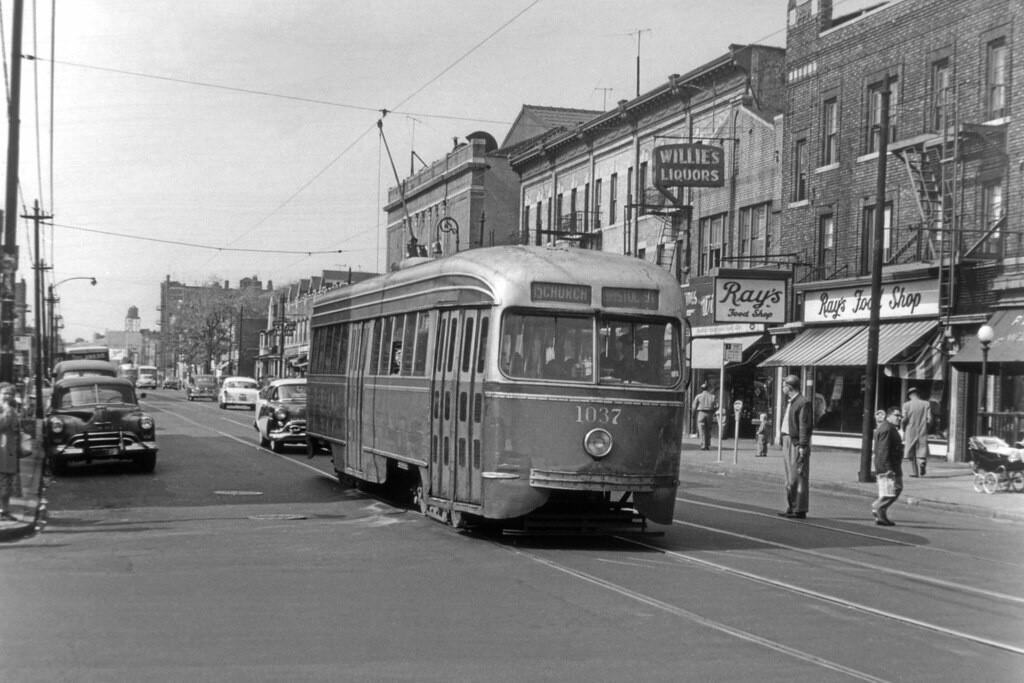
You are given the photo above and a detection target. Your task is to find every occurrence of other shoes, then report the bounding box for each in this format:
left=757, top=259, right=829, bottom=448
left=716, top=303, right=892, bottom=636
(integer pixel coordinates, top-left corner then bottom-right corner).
left=779, top=511, right=792, bottom=517
left=786, top=512, right=805, bottom=519
left=876, top=517, right=895, bottom=527
left=872, top=509, right=888, bottom=525
left=910, top=474, right=917, bottom=477
left=921, top=466, right=925, bottom=475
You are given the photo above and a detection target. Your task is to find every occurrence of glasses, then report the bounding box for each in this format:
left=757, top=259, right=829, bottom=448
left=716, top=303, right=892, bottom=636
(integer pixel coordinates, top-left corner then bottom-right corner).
left=888, top=413, right=902, bottom=418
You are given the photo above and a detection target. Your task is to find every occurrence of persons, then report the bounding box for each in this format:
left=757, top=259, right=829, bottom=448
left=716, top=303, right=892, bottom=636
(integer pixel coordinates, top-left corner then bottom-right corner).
left=871, top=406, right=905, bottom=525
left=756, top=412, right=769, bottom=456
left=777, top=374, right=813, bottom=519
left=692, top=383, right=715, bottom=449
left=902, top=387, right=932, bottom=476
left=392, top=343, right=402, bottom=376
left=615, top=333, right=653, bottom=382
left=0, top=377, right=36, bottom=514
left=714, top=385, right=730, bottom=437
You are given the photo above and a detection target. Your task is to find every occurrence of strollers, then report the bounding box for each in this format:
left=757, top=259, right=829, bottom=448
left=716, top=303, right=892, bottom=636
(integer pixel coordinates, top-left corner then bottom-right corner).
left=968, top=435, right=1024, bottom=495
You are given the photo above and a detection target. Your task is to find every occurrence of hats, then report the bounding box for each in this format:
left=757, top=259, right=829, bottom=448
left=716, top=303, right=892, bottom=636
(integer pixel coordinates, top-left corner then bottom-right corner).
left=619, top=333, right=643, bottom=350
left=906, top=387, right=921, bottom=400
left=783, top=375, right=802, bottom=389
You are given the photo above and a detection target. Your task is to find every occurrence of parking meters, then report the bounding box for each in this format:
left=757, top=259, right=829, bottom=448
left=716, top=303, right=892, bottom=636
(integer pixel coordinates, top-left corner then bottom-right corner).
left=733, top=400, right=743, bottom=464
left=876, top=409, right=886, bottom=433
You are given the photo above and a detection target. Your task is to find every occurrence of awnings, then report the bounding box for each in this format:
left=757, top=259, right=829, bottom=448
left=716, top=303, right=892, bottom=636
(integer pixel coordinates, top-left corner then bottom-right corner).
left=664, top=335, right=763, bottom=369
left=948, top=308, right=1023, bottom=375
left=757, top=320, right=938, bottom=367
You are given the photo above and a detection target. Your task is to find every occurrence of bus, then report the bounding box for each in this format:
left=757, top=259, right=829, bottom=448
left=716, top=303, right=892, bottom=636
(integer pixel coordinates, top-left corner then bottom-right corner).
left=305, top=242, right=693, bottom=539
left=139, top=366, right=157, bottom=375
left=60, top=346, right=111, bottom=361
left=119, top=365, right=138, bottom=381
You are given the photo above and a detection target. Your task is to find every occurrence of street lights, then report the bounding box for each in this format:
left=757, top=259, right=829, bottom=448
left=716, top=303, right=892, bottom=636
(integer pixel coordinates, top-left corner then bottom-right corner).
left=978, top=326, right=993, bottom=431
left=433, top=218, right=460, bottom=255
left=48, top=277, right=98, bottom=373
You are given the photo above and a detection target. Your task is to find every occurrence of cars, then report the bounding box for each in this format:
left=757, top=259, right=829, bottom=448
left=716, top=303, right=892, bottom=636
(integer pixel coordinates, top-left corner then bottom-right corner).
left=162, top=376, right=180, bottom=390
left=137, top=374, right=157, bottom=389
left=44, top=361, right=117, bottom=401
left=185, top=374, right=219, bottom=402
left=42, top=378, right=156, bottom=475
left=218, top=376, right=261, bottom=409
left=254, top=378, right=307, bottom=449
